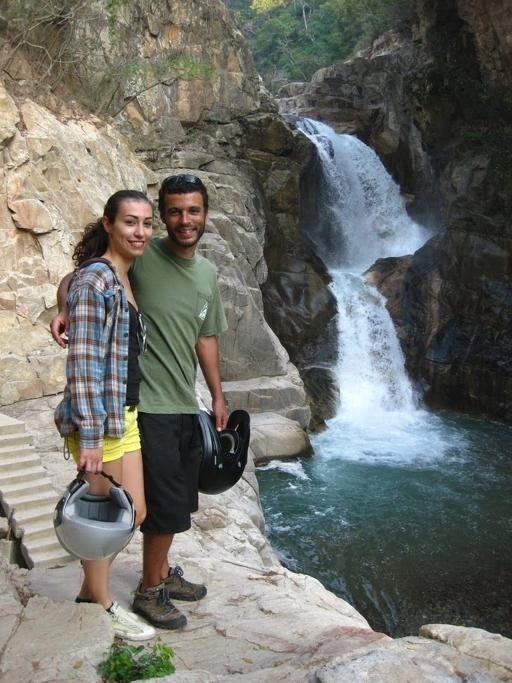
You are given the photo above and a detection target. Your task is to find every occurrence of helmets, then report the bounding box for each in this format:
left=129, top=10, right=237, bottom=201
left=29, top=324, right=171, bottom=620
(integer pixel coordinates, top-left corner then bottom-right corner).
left=53, top=478, right=137, bottom=559
left=198, top=410, right=252, bottom=495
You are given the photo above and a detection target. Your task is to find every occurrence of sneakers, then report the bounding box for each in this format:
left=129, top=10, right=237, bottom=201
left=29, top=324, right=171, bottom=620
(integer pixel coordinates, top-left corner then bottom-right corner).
left=73, top=566, right=207, bottom=645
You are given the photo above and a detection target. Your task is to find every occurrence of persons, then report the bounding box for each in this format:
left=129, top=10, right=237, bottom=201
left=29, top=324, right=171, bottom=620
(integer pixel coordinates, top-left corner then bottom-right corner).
left=53, top=190, right=155, bottom=641
left=51, top=173, right=228, bottom=630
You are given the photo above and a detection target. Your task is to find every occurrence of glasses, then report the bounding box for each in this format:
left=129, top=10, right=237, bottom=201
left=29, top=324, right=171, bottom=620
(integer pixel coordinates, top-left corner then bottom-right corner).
left=162, top=174, right=202, bottom=185
